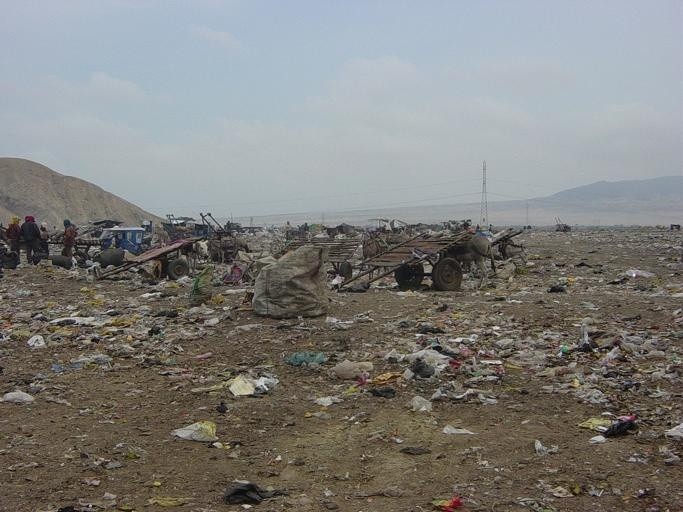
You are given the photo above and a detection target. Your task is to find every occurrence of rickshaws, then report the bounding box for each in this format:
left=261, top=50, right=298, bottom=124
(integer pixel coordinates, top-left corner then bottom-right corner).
left=95, top=237, right=204, bottom=282
left=341, top=230, right=471, bottom=293
left=274, top=237, right=365, bottom=279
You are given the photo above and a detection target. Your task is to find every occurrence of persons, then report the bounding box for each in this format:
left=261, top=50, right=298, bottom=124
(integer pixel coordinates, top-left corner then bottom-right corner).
left=475, top=224, right=481, bottom=232
left=53, top=225, right=60, bottom=244
left=463, top=222, right=470, bottom=231
left=285, top=220, right=293, bottom=240
left=4, top=214, right=50, bottom=264
left=110, top=223, right=125, bottom=238
left=304, top=222, right=310, bottom=232
left=488, top=224, right=493, bottom=232
left=62, top=220, right=76, bottom=257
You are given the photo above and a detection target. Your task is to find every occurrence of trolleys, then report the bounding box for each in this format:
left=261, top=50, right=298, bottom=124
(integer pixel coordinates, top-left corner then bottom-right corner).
left=491, top=227, right=523, bottom=259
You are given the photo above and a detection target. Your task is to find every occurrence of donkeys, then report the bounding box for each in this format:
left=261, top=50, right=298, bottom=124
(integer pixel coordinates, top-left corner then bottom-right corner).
left=440, top=235, right=497, bottom=290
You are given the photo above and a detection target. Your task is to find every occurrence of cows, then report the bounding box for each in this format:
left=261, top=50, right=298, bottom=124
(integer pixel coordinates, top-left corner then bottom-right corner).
left=179, top=240, right=210, bottom=273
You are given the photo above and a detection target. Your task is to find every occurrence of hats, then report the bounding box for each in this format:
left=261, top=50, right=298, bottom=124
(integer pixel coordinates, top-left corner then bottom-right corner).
left=12, top=216, right=47, bottom=227
